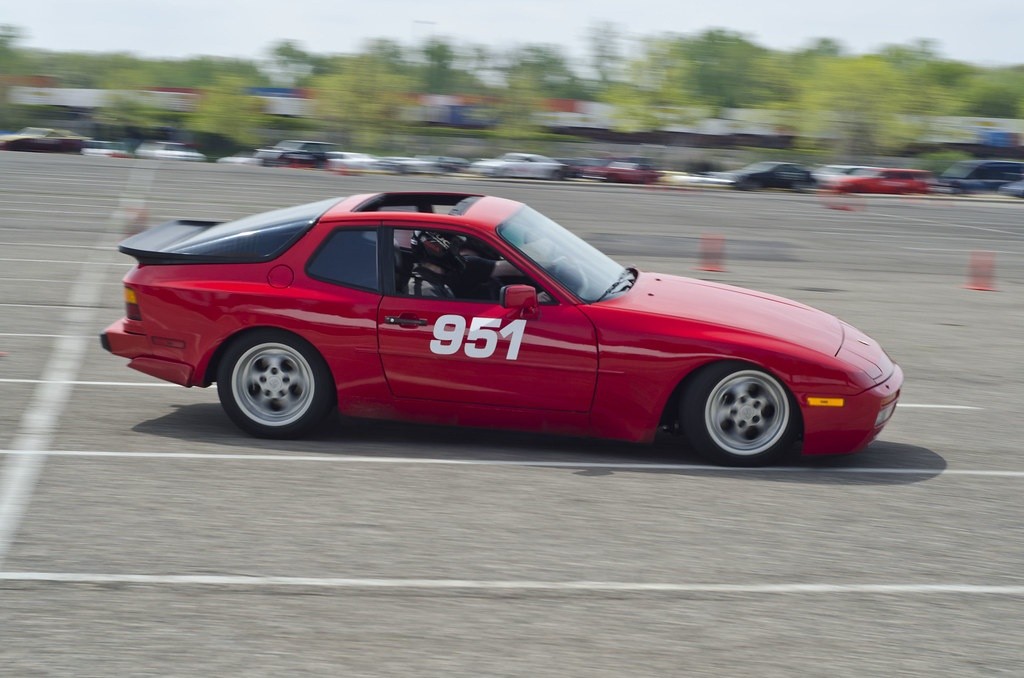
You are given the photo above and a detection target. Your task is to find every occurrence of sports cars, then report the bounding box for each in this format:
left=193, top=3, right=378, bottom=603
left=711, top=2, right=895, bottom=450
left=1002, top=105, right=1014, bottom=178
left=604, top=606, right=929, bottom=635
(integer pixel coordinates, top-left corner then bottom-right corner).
left=97, top=189, right=904, bottom=469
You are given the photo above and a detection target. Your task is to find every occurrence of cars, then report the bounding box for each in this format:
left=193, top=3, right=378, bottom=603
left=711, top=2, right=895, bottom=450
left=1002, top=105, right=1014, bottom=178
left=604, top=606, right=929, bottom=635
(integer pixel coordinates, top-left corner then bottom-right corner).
left=83, top=139, right=1024, bottom=203
left=0, top=126, right=90, bottom=156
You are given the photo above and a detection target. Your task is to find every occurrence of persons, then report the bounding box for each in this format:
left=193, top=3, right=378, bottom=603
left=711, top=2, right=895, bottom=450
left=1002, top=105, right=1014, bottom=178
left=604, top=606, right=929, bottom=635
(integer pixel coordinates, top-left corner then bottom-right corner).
left=449, top=231, right=564, bottom=305
left=406, top=228, right=551, bottom=305
